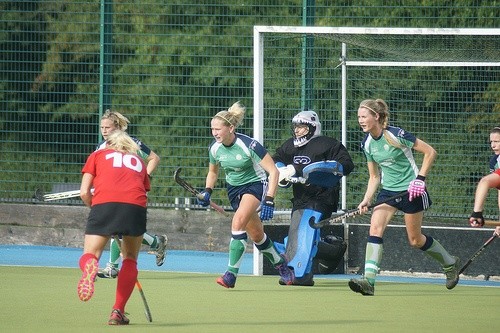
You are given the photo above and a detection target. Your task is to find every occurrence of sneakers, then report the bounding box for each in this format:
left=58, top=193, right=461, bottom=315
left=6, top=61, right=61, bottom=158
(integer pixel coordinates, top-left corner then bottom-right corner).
left=274, top=253, right=295, bottom=285
left=78, top=258, right=99, bottom=301
left=217, top=271, right=236, bottom=288
left=108, top=310, right=130, bottom=325
left=148, top=234, right=167, bottom=267
left=348, top=279, right=374, bottom=295
left=443, top=257, right=461, bottom=289
left=97, top=265, right=121, bottom=278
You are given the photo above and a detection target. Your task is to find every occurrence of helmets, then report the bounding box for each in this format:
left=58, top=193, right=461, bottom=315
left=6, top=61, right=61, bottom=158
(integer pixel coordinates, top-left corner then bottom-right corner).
left=290, top=110, right=322, bottom=148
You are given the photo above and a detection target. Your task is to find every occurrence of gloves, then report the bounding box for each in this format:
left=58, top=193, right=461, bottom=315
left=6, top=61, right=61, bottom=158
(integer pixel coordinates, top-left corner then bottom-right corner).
left=407, top=175, right=425, bottom=202
left=197, top=188, right=213, bottom=207
left=256, top=196, right=274, bottom=221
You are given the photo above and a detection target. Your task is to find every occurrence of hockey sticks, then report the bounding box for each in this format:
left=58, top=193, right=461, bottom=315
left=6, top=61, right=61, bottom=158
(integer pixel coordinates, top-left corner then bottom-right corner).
left=174, top=168, right=231, bottom=217
left=114, top=235, right=153, bottom=323
left=459, top=233, right=497, bottom=275
left=308, top=189, right=410, bottom=229
left=34, top=187, right=94, bottom=201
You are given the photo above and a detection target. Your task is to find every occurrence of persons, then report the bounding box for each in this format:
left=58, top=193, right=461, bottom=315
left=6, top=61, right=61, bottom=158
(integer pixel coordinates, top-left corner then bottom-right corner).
left=468, top=169, right=500, bottom=228
left=489, top=126, right=500, bottom=174
left=197, top=101, right=295, bottom=288
left=77, top=130, right=150, bottom=327
left=348, top=98, right=460, bottom=295
left=271, top=111, right=354, bottom=286
left=97, top=110, right=167, bottom=278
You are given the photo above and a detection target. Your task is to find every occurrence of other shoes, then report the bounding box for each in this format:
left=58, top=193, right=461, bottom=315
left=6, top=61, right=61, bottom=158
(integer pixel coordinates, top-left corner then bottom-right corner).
left=279, top=274, right=314, bottom=285
